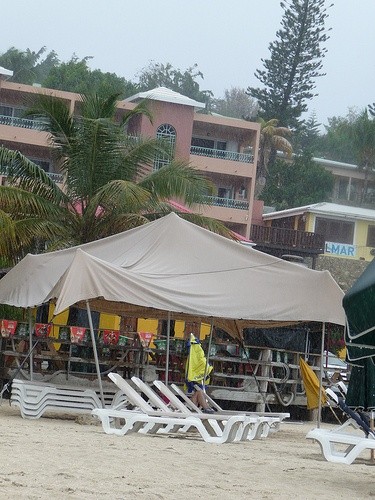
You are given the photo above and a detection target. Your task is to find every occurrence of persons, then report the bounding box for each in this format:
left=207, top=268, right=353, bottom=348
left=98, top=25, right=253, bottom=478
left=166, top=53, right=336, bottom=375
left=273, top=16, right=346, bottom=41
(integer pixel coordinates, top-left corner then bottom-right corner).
left=150, top=383, right=217, bottom=414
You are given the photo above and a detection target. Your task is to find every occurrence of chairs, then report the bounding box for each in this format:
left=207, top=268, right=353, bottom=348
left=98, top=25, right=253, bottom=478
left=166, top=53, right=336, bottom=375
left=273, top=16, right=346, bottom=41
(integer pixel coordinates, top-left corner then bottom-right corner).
left=90, top=372, right=291, bottom=445
left=305, top=380, right=375, bottom=465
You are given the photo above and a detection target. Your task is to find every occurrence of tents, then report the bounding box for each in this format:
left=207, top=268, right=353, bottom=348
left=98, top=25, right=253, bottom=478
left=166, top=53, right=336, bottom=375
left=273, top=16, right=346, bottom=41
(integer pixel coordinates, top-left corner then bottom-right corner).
left=0, top=211, right=348, bottom=432
